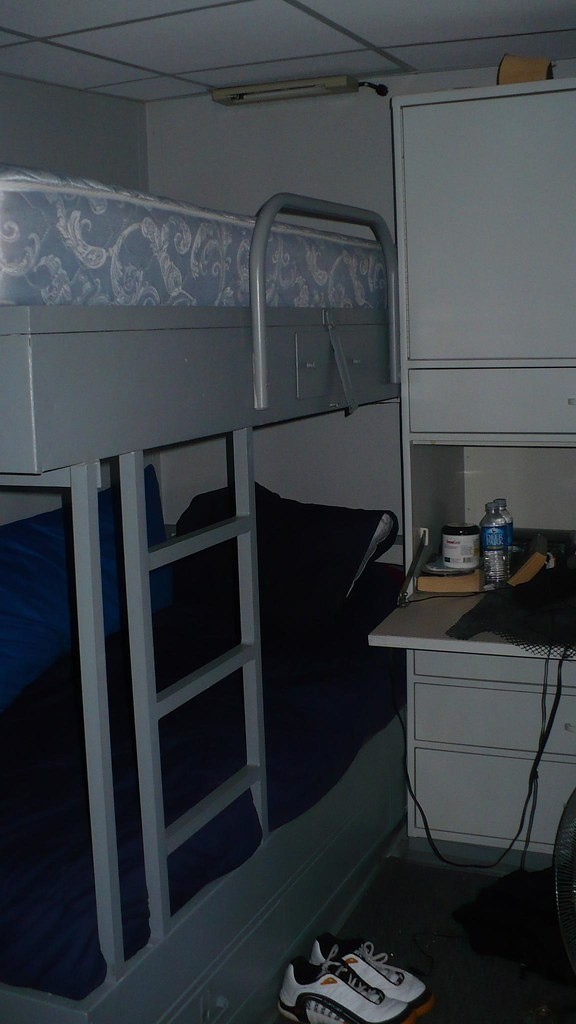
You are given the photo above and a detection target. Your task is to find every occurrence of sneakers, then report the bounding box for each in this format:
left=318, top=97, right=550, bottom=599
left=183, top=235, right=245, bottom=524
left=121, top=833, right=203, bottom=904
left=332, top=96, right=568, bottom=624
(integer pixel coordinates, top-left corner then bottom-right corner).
left=309, top=931, right=434, bottom=1016
left=278, top=955, right=416, bottom=1024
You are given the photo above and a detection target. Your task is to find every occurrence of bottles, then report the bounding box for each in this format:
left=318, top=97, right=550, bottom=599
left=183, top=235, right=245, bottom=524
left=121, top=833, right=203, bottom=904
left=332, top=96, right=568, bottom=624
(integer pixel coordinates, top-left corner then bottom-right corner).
left=493, top=499, right=513, bottom=578
left=480, top=502, right=508, bottom=586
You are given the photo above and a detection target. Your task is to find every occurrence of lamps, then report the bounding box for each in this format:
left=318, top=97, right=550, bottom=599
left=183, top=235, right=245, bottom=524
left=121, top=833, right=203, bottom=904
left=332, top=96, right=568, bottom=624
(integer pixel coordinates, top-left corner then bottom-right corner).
left=211, top=75, right=388, bottom=106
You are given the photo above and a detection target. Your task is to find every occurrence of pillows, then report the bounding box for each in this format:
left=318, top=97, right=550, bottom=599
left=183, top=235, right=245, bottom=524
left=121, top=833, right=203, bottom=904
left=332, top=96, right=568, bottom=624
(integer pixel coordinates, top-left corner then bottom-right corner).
left=0, top=462, right=173, bottom=716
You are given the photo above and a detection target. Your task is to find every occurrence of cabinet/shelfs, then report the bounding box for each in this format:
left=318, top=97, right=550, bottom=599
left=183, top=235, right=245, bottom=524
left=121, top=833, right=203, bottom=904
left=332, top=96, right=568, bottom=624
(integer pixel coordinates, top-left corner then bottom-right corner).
left=365, top=78, right=576, bottom=857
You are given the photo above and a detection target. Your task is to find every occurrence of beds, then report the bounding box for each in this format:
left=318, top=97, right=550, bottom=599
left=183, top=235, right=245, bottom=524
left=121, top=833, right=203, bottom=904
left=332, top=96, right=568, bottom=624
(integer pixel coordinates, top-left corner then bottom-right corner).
left=0, top=164, right=411, bottom=1024
